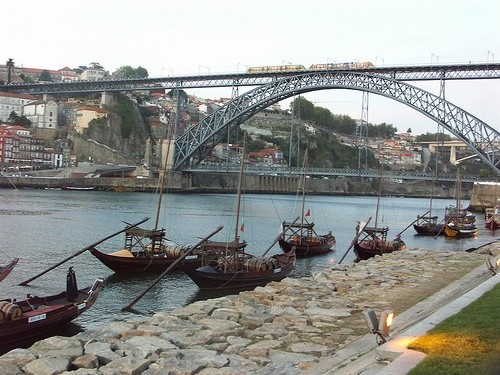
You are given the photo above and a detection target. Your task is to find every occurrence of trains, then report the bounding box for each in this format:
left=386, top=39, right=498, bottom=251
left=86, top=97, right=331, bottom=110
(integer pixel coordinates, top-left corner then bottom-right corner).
left=248, top=62, right=374, bottom=73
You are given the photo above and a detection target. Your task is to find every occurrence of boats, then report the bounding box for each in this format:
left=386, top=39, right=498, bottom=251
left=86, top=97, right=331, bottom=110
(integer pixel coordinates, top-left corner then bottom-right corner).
left=0, top=248, right=105, bottom=353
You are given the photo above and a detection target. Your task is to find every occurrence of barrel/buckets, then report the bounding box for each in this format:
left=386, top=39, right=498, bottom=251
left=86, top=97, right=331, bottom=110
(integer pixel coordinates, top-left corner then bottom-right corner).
left=311, top=238, right=321, bottom=246
left=383, top=240, right=395, bottom=251
left=146, top=244, right=164, bottom=252
left=180, top=244, right=198, bottom=255
left=243, top=256, right=279, bottom=272
left=0, top=301, right=23, bottom=323
left=166, top=246, right=186, bottom=257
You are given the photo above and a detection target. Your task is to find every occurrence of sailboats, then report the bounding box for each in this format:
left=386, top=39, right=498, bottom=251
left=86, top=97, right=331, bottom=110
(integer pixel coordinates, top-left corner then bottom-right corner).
left=413, top=169, right=500, bottom=238
left=278, top=164, right=336, bottom=258
left=353, top=153, right=406, bottom=262
left=181, top=128, right=296, bottom=292
left=88, top=136, right=242, bottom=277
